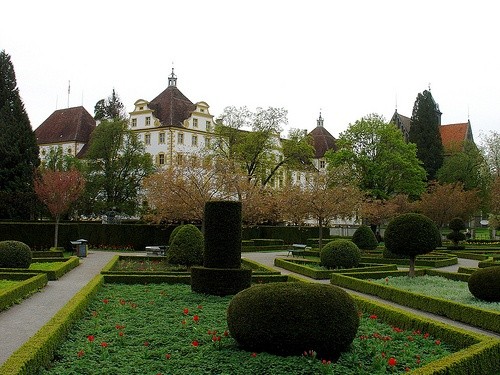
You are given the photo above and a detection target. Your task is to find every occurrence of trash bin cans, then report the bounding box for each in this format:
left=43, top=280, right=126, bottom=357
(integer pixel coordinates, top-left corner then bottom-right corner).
left=77, top=239, right=88, bottom=257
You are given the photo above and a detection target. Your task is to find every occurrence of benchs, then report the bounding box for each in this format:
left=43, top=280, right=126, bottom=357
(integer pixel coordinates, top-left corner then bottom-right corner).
left=70, top=239, right=82, bottom=256
left=287, top=243, right=307, bottom=259
